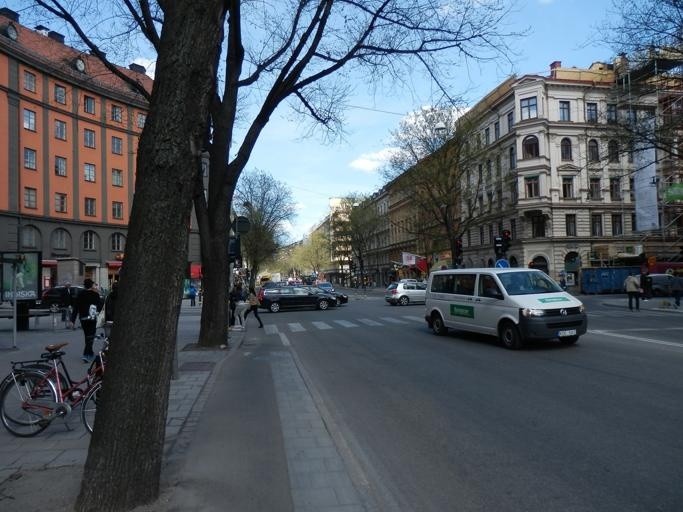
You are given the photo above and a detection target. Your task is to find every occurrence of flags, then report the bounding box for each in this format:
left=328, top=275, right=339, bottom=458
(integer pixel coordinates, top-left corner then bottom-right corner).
left=415, top=257, right=428, bottom=274
left=402, top=253, right=416, bottom=266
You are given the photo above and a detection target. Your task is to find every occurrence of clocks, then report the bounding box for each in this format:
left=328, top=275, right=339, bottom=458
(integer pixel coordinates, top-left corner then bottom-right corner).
left=7, top=23, right=18, bottom=40
left=76, top=57, right=85, bottom=73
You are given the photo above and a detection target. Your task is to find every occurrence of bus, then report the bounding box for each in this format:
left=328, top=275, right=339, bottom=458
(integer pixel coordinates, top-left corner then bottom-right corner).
left=640, top=251, right=683, bottom=274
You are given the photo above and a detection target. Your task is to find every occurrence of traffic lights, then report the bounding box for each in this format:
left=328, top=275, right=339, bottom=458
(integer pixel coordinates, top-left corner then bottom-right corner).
left=503, top=230, right=512, bottom=250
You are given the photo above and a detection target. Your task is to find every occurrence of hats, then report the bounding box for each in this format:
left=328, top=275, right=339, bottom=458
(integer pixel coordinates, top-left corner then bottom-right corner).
left=82, top=278, right=93, bottom=285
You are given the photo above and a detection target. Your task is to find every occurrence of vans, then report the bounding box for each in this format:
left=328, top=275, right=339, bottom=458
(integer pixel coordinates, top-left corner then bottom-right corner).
left=259, top=286, right=337, bottom=313
left=425, top=267, right=588, bottom=350
left=384, top=281, right=427, bottom=306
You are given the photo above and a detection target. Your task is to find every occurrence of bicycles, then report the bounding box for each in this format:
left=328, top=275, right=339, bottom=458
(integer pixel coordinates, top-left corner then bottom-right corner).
left=1, top=333, right=109, bottom=438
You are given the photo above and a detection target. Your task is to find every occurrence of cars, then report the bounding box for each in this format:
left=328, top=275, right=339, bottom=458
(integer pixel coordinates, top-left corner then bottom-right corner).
left=636, top=274, right=683, bottom=297
left=42, top=285, right=105, bottom=313
left=330, top=293, right=348, bottom=307
left=318, top=282, right=335, bottom=292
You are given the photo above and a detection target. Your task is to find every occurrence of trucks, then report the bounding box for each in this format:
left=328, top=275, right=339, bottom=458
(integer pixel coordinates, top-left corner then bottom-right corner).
left=260, top=274, right=272, bottom=286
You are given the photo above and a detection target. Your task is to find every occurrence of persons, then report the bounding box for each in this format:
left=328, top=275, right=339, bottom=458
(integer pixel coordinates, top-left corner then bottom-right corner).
left=71, top=279, right=103, bottom=363
left=188, top=284, right=196, bottom=307
left=58, top=282, right=73, bottom=321
left=103, top=281, right=117, bottom=348
left=624, top=271, right=641, bottom=312
left=670, top=270, right=683, bottom=308
left=557, top=273, right=566, bottom=292
left=243, top=286, right=263, bottom=328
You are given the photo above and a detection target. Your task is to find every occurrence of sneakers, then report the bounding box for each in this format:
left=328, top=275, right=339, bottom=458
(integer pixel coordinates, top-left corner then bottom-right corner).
left=80, top=354, right=96, bottom=364
